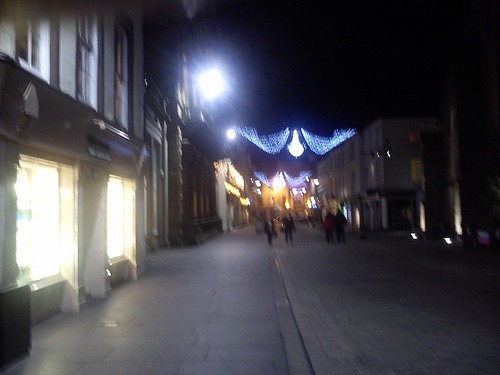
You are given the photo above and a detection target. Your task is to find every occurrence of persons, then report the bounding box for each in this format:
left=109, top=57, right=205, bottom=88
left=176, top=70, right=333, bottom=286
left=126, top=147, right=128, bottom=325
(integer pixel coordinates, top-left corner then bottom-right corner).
left=263, top=204, right=350, bottom=246
